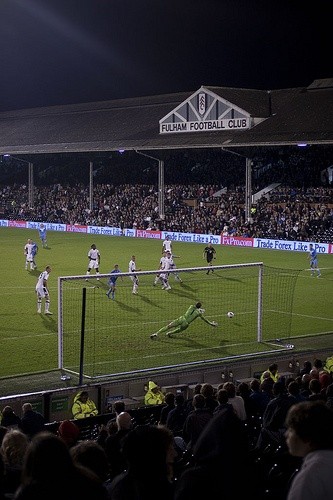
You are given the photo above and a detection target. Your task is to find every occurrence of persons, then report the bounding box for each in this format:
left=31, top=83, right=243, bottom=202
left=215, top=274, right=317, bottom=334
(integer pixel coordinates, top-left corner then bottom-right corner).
left=106, top=264, right=124, bottom=299
left=154, top=253, right=183, bottom=290
left=161, top=236, right=174, bottom=255
left=38, top=224, right=48, bottom=248
left=0, top=183, right=333, bottom=244
left=308, top=244, right=321, bottom=277
left=24, top=239, right=38, bottom=271
left=35, top=265, right=54, bottom=315
left=150, top=302, right=218, bottom=338
left=85, top=244, right=100, bottom=282
left=203, top=242, right=217, bottom=275
left=0, top=355, right=333, bottom=500
left=129, top=256, right=142, bottom=295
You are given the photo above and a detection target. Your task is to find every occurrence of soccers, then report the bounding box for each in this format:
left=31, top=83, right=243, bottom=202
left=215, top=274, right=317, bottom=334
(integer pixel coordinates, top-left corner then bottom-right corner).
left=227, top=312, right=234, bottom=318
